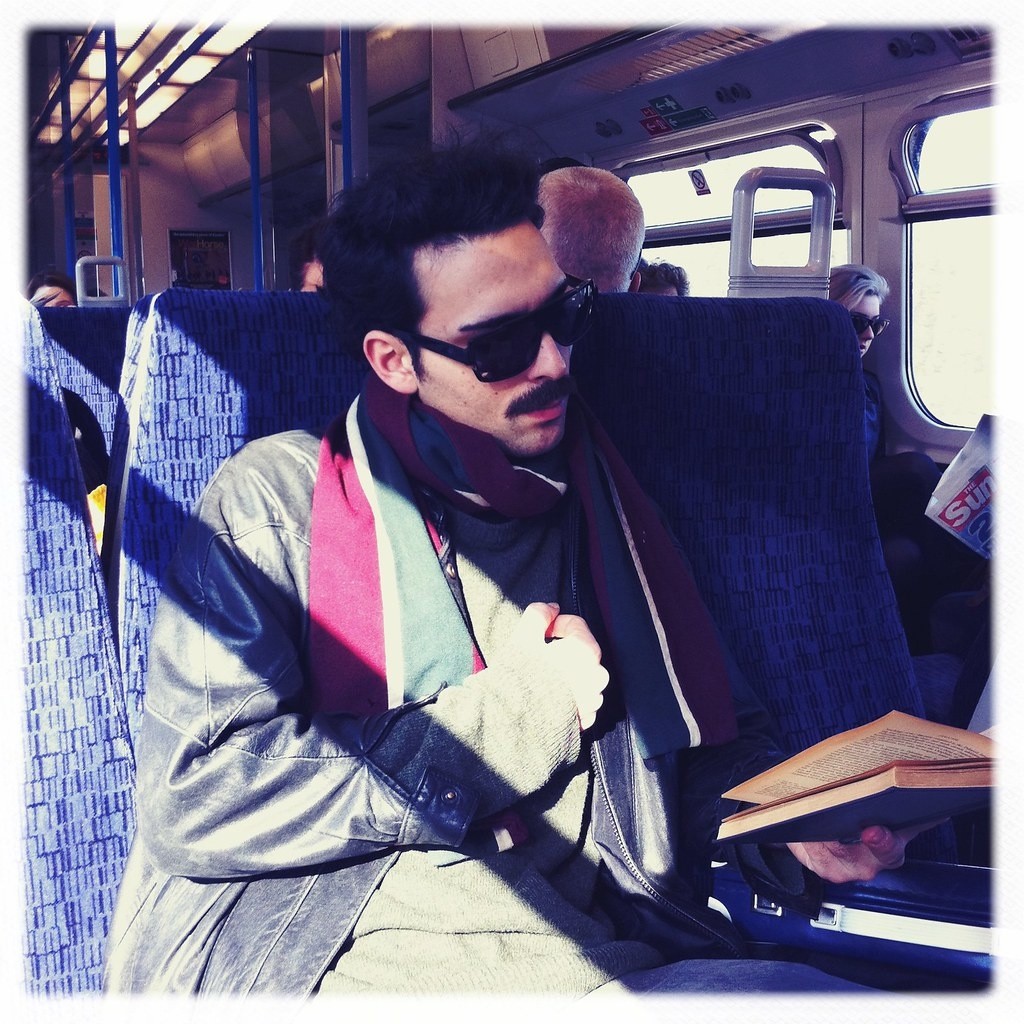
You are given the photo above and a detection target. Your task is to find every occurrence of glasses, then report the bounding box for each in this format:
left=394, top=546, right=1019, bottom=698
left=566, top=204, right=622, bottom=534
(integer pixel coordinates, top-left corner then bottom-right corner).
left=849, top=310, right=887, bottom=339
left=407, top=275, right=600, bottom=383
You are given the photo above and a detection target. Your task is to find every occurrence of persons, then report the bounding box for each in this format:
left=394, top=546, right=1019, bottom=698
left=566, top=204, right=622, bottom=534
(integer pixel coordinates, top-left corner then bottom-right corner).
left=828, top=263, right=942, bottom=655
left=60, top=386, right=109, bottom=555
left=638, top=263, right=689, bottom=296
left=108, top=143, right=949, bottom=994
left=537, top=167, right=645, bottom=293
left=26, top=268, right=77, bottom=308
left=287, top=222, right=324, bottom=293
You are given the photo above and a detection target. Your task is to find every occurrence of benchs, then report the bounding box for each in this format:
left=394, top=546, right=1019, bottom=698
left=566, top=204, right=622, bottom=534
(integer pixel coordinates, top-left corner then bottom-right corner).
left=26, top=289, right=994, bottom=996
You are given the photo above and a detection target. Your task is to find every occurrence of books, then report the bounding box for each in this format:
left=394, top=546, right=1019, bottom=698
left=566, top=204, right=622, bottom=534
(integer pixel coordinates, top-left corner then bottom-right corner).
left=717, top=711, right=995, bottom=847
